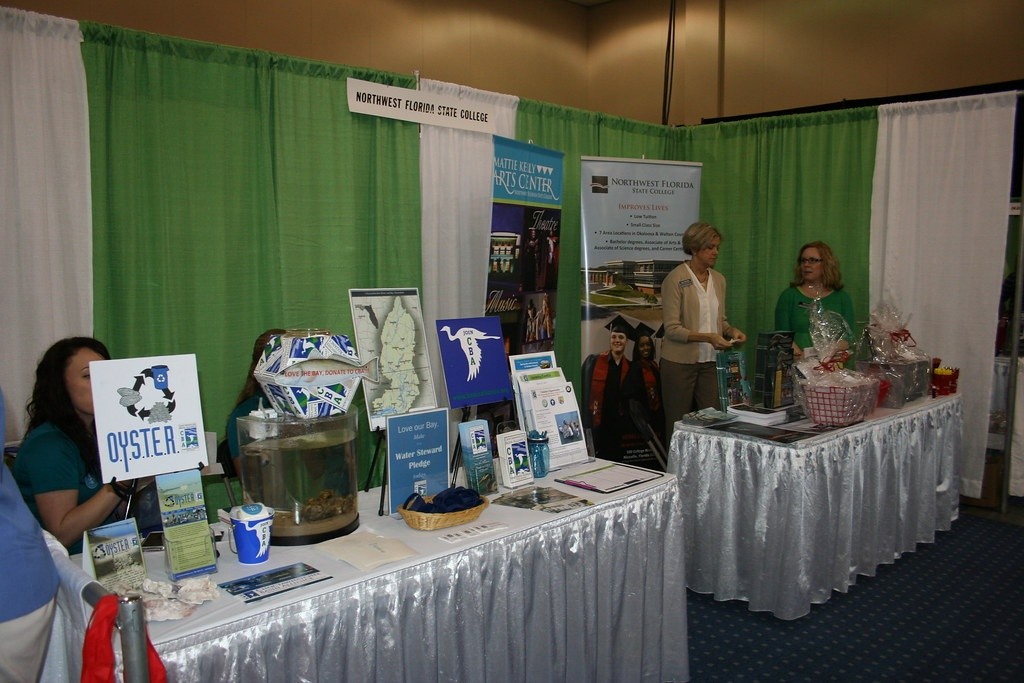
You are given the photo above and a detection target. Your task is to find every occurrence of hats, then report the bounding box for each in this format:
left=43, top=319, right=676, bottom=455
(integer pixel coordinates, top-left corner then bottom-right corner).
left=602, top=315, right=666, bottom=341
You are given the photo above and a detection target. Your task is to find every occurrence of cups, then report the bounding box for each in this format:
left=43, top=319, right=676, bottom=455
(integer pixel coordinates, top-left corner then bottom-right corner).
left=952, top=372, right=959, bottom=393
left=935, top=373, right=953, bottom=395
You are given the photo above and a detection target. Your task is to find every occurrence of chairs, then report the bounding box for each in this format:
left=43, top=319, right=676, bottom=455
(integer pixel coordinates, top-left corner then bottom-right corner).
left=217, top=439, right=240, bottom=508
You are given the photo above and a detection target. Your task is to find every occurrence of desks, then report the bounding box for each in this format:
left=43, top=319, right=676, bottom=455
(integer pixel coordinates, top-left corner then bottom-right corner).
left=665, top=392, right=966, bottom=621
left=70, top=458, right=691, bottom=683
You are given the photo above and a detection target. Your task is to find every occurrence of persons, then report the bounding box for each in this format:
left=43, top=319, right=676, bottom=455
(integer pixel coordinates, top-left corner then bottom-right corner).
left=488, top=227, right=561, bottom=291
left=0, top=338, right=133, bottom=683
left=226, top=328, right=350, bottom=511
left=659, top=222, right=747, bottom=453
left=775, top=241, right=855, bottom=373
left=582, top=316, right=667, bottom=464
left=524, top=292, right=554, bottom=343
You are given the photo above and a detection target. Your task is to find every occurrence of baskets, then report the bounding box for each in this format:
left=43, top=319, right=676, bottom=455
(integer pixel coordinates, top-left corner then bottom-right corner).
left=397, top=492, right=490, bottom=532
left=801, top=379, right=879, bottom=427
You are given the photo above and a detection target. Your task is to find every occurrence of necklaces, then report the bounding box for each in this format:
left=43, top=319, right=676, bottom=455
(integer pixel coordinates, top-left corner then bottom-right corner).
left=689, top=261, right=708, bottom=283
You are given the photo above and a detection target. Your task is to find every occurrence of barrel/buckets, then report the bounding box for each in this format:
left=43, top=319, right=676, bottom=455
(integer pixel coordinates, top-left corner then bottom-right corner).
left=228, top=507, right=275, bottom=565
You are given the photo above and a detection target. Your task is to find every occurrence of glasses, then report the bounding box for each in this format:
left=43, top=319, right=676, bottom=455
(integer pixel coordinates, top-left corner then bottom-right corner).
left=800, top=257, right=823, bottom=264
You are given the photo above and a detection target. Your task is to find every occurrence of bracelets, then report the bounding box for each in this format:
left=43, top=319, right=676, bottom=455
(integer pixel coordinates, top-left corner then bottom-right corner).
left=120, top=481, right=130, bottom=488
left=724, top=327, right=733, bottom=338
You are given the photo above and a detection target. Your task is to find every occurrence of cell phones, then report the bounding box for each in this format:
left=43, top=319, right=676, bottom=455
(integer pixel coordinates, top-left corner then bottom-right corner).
left=140, top=531, right=164, bottom=550
